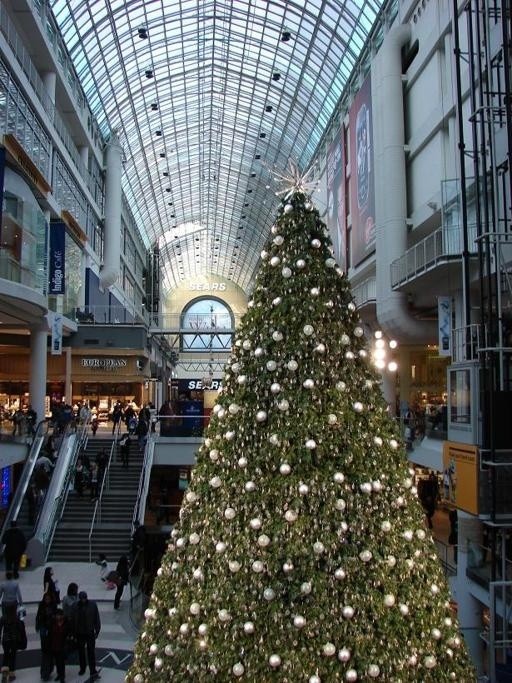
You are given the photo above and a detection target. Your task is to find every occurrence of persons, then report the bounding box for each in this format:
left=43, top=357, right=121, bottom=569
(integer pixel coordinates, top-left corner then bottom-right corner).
left=74, top=447, right=110, bottom=501
left=392, top=393, right=457, bottom=562
left=3, top=567, right=102, bottom=681
left=95, top=554, right=110, bottom=585
left=76, top=307, right=94, bottom=319
left=91, top=419, right=99, bottom=436
left=12, top=400, right=81, bottom=505
left=111, top=399, right=169, bottom=467
left=114, top=520, right=147, bottom=609
left=3, top=521, right=27, bottom=580
left=62, top=306, right=76, bottom=319
